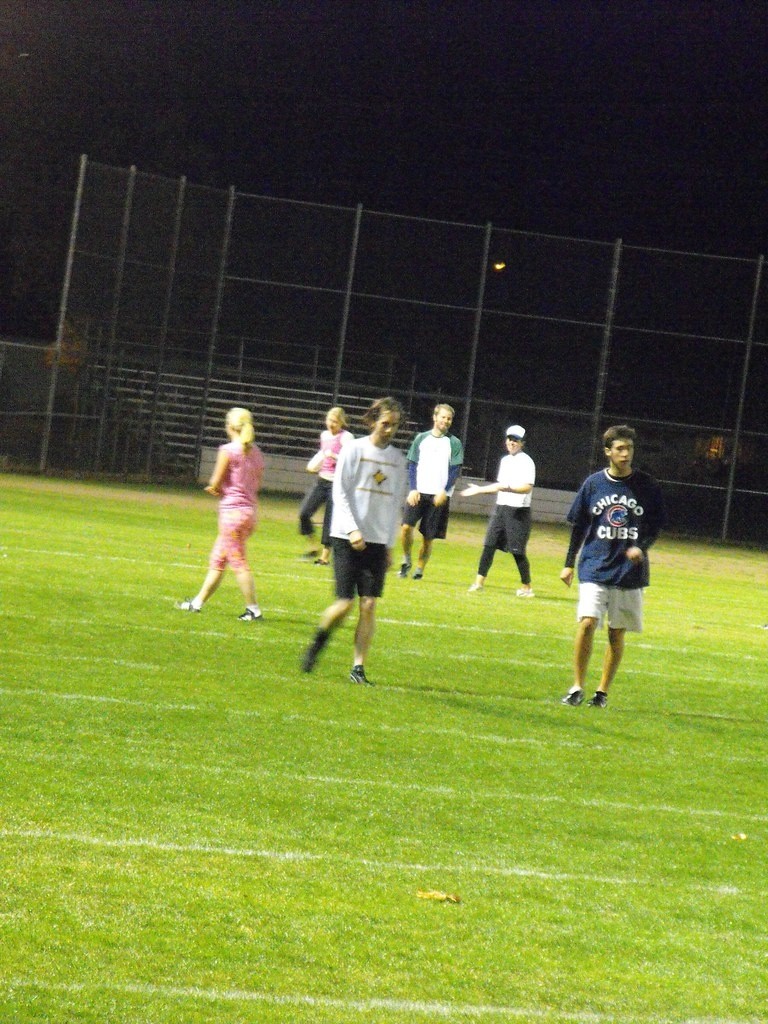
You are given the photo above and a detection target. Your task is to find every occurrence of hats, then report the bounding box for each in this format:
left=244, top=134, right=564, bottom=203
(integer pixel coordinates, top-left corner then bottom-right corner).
left=504, top=425, right=527, bottom=441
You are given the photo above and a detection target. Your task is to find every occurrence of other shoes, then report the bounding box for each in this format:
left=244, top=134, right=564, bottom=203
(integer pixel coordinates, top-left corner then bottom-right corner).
left=350, top=670, right=371, bottom=686
left=236, top=608, right=264, bottom=621
left=302, top=549, right=319, bottom=558
left=467, top=585, right=484, bottom=594
left=313, top=559, right=330, bottom=565
left=515, top=587, right=535, bottom=598
left=412, top=573, right=423, bottom=580
left=301, top=647, right=314, bottom=672
left=397, top=563, right=412, bottom=577
left=174, top=596, right=201, bottom=612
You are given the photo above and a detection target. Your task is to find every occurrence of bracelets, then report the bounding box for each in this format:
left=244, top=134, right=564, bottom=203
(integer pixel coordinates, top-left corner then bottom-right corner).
left=506, top=483, right=511, bottom=492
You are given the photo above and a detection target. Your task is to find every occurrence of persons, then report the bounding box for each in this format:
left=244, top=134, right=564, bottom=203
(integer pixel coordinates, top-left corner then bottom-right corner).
left=303, top=397, right=410, bottom=687
left=398, top=403, right=464, bottom=579
left=460, top=425, right=538, bottom=597
left=175, top=407, right=263, bottom=621
left=560, top=426, right=666, bottom=706
left=298, top=407, right=356, bottom=565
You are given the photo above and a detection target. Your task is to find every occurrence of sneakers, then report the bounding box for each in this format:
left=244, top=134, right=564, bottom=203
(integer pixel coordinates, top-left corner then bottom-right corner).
left=586, top=691, right=608, bottom=708
left=562, top=687, right=585, bottom=706
